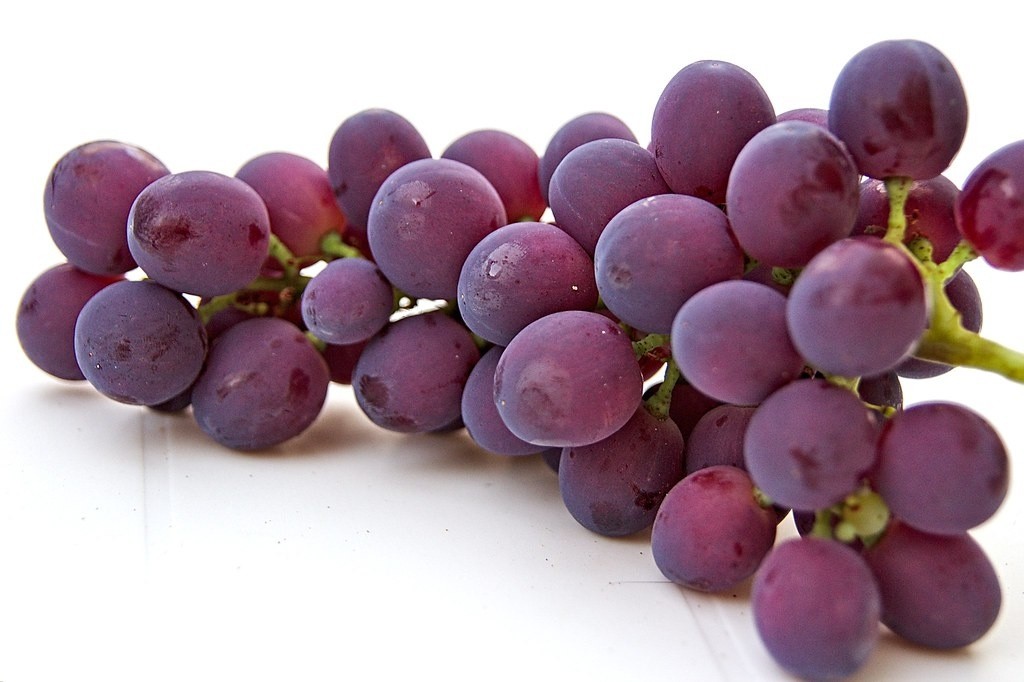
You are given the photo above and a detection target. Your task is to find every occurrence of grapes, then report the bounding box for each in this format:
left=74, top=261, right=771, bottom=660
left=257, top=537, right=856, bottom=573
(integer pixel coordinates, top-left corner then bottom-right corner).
left=17, top=39, right=1024, bottom=682
